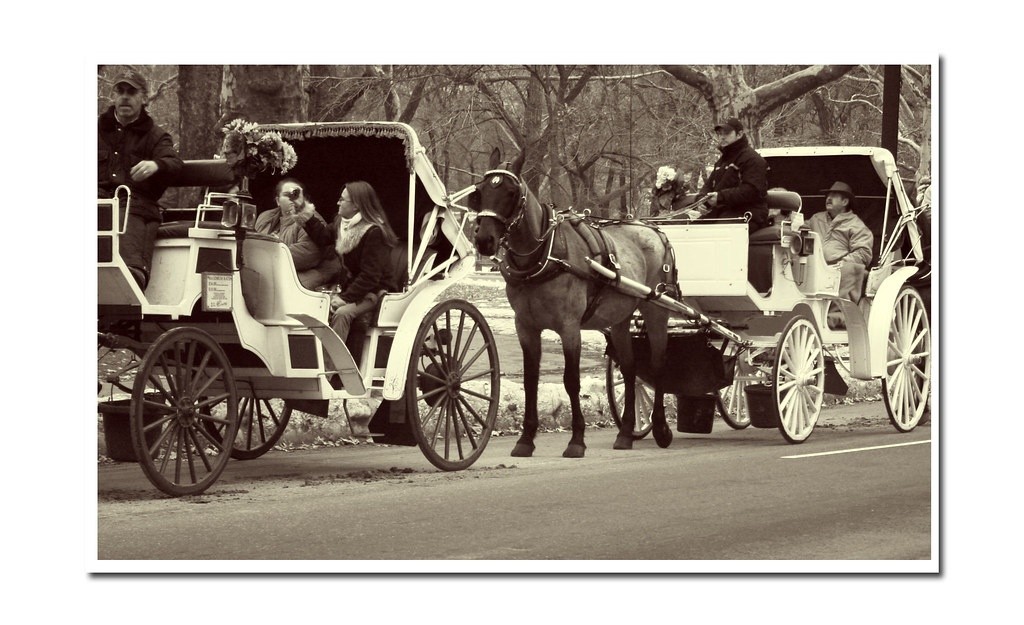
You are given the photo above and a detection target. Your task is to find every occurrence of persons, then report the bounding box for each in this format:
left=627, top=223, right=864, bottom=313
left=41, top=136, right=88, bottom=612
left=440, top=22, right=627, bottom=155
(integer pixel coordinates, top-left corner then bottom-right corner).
left=804, top=181, right=873, bottom=271
left=255, top=179, right=399, bottom=382
left=98, top=73, right=184, bottom=293
left=689, top=117, right=769, bottom=237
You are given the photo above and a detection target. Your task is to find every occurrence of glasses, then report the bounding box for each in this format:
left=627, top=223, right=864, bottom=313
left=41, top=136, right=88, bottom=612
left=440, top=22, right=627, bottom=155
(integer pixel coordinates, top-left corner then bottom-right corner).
left=340, top=196, right=352, bottom=204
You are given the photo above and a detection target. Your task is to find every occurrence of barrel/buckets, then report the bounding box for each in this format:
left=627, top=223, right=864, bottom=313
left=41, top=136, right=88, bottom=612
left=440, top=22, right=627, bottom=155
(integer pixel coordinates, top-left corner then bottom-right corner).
left=99, top=402, right=162, bottom=460
left=744, top=384, right=777, bottom=428
left=677, top=395, right=716, bottom=433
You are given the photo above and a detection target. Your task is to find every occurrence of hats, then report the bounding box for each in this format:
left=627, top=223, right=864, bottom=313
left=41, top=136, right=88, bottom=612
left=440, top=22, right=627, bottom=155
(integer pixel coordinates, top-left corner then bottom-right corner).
left=821, top=181, right=853, bottom=202
left=113, top=70, right=148, bottom=93
left=714, top=117, right=745, bottom=132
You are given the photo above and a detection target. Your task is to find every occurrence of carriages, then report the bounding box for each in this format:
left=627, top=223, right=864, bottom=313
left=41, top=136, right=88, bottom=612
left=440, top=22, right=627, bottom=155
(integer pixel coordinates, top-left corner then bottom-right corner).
left=464, top=143, right=930, bottom=444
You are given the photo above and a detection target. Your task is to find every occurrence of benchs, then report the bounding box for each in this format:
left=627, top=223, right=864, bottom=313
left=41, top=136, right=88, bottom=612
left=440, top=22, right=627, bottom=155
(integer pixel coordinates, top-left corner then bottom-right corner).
left=666, top=191, right=803, bottom=245
left=320, top=242, right=439, bottom=329
left=247, top=233, right=332, bottom=330
left=801, top=231, right=841, bottom=296
left=154, top=158, right=234, bottom=249
left=862, top=218, right=894, bottom=296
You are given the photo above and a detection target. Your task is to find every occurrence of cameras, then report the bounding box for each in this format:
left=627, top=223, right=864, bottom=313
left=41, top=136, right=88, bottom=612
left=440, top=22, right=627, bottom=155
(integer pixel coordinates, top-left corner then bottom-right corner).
left=288, top=188, right=300, bottom=201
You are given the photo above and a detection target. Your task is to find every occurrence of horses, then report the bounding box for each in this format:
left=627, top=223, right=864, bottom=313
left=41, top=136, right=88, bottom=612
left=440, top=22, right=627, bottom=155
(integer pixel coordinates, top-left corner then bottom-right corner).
left=466, top=147, right=678, bottom=458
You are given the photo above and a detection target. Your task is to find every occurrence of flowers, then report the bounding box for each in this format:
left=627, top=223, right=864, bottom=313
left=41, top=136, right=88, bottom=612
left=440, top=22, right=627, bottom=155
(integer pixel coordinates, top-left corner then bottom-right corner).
left=219, top=118, right=298, bottom=183
left=651, top=166, right=690, bottom=208
